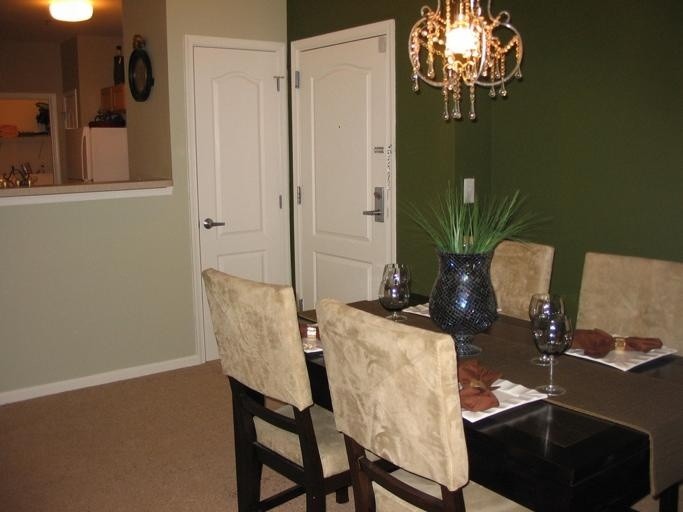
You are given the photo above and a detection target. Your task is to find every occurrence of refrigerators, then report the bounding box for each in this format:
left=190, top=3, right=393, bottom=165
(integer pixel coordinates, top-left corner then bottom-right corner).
left=64, top=128, right=128, bottom=182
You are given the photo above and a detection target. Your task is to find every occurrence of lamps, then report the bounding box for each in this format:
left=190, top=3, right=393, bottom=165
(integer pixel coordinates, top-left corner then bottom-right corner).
left=405, top=0, right=524, bottom=123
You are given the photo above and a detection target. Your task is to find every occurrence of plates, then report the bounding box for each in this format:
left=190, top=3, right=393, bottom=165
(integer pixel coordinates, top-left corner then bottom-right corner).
left=563, top=336, right=679, bottom=373
left=460, top=379, right=546, bottom=423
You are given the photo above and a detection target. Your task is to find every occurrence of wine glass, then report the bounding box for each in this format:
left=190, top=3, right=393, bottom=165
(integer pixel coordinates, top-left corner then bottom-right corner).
left=529, top=294, right=573, bottom=395
left=377, top=263, right=409, bottom=323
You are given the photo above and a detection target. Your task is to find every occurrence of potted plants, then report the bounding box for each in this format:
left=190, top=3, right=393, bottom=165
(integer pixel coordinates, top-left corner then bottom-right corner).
left=405, top=184, right=542, bottom=334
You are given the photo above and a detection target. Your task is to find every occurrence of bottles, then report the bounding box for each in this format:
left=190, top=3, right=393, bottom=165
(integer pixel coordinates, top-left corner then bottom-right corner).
left=114, top=44, right=124, bottom=84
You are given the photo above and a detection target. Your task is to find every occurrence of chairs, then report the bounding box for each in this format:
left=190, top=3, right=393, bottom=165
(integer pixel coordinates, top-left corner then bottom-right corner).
left=487, top=238, right=683, bottom=350
left=198, top=267, right=401, bottom=512
left=312, top=294, right=533, bottom=512
